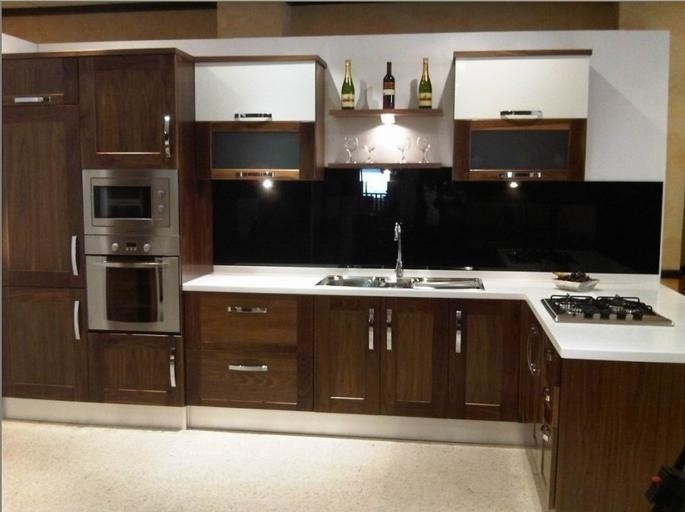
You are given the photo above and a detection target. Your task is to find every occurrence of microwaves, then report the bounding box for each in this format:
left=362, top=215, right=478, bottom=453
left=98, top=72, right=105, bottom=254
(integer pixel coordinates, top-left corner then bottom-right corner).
left=82, top=168, right=180, bottom=236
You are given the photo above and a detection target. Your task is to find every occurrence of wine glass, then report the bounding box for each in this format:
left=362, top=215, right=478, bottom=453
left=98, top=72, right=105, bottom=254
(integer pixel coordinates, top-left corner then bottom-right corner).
left=343, top=136, right=431, bottom=164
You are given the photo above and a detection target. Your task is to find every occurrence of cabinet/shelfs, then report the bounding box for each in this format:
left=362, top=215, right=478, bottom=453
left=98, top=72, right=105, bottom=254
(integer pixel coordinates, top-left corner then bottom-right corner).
left=526, top=301, right=684, bottom=510
left=183, top=291, right=525, bottom=425
left=452, top=50, right=592, bottom=182
left=194, top=55, right=327, bottom=182
left=328, top=108, right=442, bottom=169
left=2, top=47, right=213, bottom=406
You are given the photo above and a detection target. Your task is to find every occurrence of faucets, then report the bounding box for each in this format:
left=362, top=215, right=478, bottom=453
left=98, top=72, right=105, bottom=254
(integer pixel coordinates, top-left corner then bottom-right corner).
left=393, top=221, right=403, bottom=277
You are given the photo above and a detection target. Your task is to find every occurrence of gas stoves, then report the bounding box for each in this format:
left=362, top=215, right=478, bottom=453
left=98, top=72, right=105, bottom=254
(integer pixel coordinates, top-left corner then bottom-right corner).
left=541, top=293, right=676, bottom=327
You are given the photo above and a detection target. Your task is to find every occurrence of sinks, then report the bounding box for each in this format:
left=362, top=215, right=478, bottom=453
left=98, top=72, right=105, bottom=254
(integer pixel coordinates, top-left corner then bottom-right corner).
left=424, top=277, right=485, bottom=290
left=369, top=276, right=424, bottom=289
left=317, top=275, right=369, bottom=287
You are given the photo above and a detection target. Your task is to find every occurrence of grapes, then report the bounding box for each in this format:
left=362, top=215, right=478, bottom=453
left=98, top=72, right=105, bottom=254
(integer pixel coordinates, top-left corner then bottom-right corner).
left=568, top=272, right=591, bottom=281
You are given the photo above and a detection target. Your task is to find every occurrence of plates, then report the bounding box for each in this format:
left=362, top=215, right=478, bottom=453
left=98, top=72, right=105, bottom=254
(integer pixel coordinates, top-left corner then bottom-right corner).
left=552, top=279, right=601, bottom=291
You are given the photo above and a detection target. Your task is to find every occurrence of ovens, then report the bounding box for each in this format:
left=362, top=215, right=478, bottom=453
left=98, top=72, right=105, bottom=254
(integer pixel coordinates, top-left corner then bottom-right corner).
left=85, top=236, right=180, bottom=335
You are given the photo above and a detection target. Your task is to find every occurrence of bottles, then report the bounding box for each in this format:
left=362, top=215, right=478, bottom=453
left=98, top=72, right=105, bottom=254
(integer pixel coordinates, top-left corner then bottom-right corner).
left=339, top=59, right=355, bottom=110
left=418, top=56, right=433, bottom=110
left=382, top=62, right=396, bottom=110
